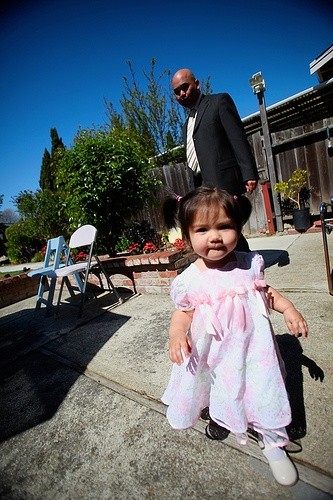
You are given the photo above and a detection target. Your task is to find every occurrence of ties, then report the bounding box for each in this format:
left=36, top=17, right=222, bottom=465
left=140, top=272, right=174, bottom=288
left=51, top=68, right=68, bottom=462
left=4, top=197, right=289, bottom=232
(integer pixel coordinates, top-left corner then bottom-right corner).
left=185, top=112, right=201, bottom=172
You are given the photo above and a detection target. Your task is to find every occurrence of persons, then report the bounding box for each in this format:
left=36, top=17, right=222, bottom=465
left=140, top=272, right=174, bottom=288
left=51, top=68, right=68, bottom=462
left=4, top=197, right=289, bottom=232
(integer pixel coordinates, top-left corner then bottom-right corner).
left=159, top=187, right=310, bottom=485
left=171, top=69, right=259, bottom=252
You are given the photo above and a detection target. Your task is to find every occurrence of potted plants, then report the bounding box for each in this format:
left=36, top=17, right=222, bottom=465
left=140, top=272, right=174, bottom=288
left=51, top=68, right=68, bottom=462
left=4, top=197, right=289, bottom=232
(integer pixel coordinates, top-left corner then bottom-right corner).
left=273, top=168, right=311, bottom=230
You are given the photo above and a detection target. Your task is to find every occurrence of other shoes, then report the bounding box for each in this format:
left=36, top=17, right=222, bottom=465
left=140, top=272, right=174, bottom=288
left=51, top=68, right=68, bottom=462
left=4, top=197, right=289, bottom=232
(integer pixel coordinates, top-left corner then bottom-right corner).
left=262, top=447, right=298, bottom=486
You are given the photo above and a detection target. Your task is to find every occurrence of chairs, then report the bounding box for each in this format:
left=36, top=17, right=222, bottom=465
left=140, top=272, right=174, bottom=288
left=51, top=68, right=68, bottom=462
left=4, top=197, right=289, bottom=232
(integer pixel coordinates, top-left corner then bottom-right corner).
left=27, top=225, right=123, bottom=318
